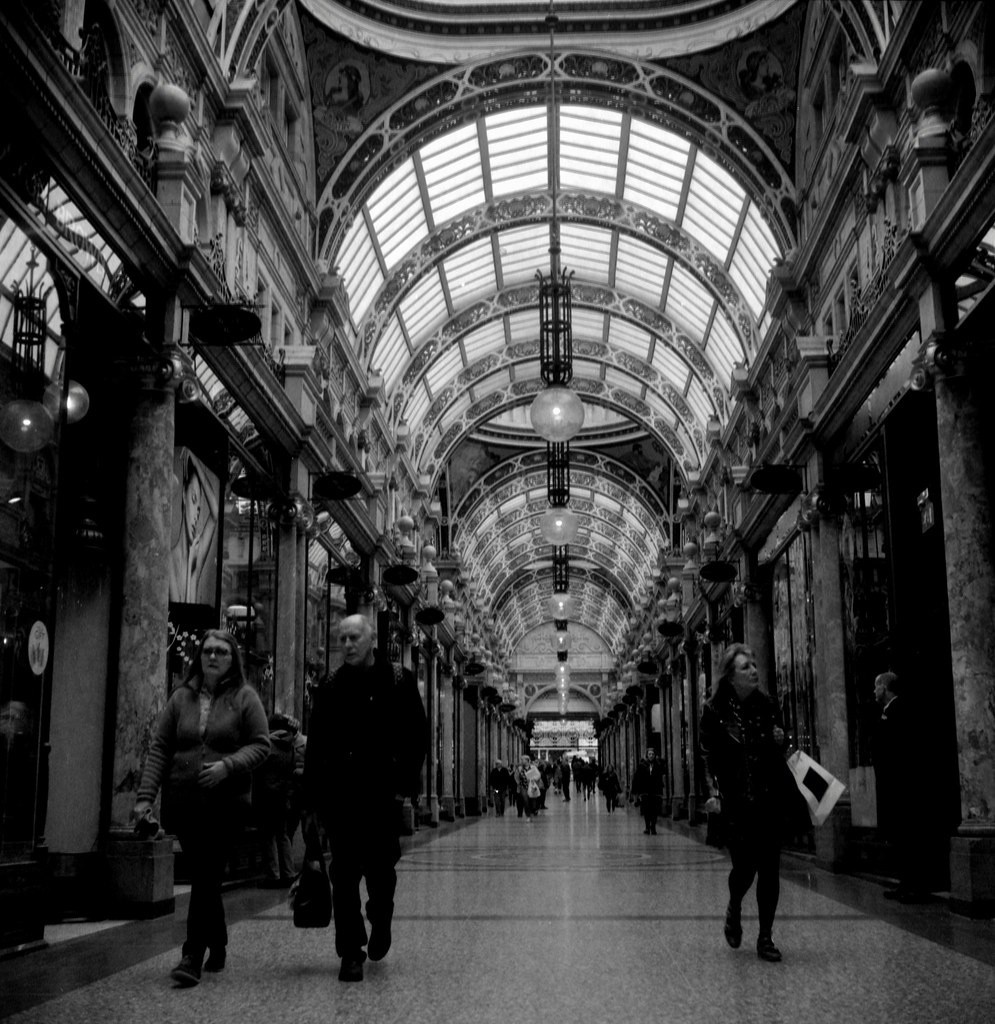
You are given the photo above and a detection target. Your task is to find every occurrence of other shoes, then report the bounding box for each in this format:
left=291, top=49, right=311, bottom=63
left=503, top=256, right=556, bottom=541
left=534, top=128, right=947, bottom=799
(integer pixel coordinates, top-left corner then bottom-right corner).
left=204, top=946, right=226, bottom=972
left=367, top=925, right=392, bottom=961
left=882, top=887, right=918, bottom=907
left=724, top=901, right=743, bottom=948
left=338, top=958, right=365, bottom=982
left=756, top=935, right=783, bottom=962
left=170, top=951, right=204, bottom=984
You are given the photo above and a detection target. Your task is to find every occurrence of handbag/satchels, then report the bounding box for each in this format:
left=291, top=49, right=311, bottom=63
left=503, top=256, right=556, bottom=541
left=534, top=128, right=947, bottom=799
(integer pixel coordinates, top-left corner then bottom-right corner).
left=785, top=749, right=846, bottom=825
left=526, top=779, right=541, bottom=799
left=287, top=840, right=332, bottom=928
left=848, top=743, right=879, bottom=828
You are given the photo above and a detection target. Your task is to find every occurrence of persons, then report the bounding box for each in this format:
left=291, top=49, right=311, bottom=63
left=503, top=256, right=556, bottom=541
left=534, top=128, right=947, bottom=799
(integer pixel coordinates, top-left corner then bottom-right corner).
left=871, top=671, right=932, bottom=906
left=699, top=643, right=791, bottom=962
left=302, top=613, right=429, bottom=982
left=632, top=748, right=665, bottom=835
left=251, top=714, right=307, bottom=885
left=129, top=630, right=272, bottom=987
left=490, top=755, right=622, bottom=823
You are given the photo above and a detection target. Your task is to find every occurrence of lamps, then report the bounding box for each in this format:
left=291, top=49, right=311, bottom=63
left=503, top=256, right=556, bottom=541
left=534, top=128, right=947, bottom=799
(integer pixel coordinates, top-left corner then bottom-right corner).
left=540, top=436, right=579, bottom=726
left=527, top=0, right=586, bottom=446
left=0, top=246, right=90, bottom=452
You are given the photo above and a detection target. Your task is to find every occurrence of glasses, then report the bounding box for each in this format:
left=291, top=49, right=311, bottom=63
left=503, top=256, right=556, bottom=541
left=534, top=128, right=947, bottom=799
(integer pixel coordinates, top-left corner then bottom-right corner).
left=200, top=647, right=232, bottom=657
